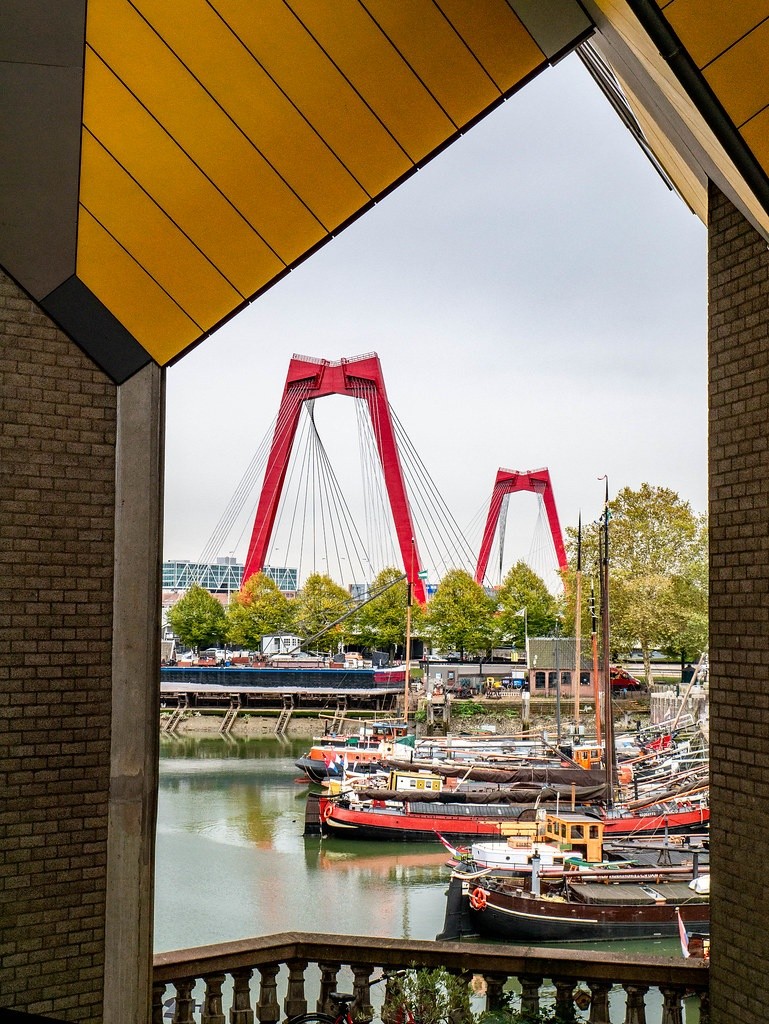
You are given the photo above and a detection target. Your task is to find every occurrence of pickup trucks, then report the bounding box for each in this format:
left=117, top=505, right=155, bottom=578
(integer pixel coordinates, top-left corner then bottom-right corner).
left=199, top=647, right=219, bottom=659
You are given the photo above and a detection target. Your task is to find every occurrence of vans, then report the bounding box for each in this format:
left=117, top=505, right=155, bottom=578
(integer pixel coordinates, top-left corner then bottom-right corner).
left=608, top=668, right=642, bottom=692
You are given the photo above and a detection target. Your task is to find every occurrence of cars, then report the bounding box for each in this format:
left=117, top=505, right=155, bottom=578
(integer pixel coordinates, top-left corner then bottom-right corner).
left=214, top=650, right=236, bottom=661
left=426, top=654, right=447, bottom=661
left=345, top=651, right=362, bottom=660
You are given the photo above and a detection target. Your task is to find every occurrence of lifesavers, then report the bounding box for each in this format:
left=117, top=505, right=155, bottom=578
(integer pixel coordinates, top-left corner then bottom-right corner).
left=470, top=888, right=487, bottom=910
left=324, top=806, right=333, bottom=818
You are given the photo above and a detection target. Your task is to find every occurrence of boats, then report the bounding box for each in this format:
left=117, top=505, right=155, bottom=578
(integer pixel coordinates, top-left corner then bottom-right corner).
left=294, top=527, right=710, bottom=960
